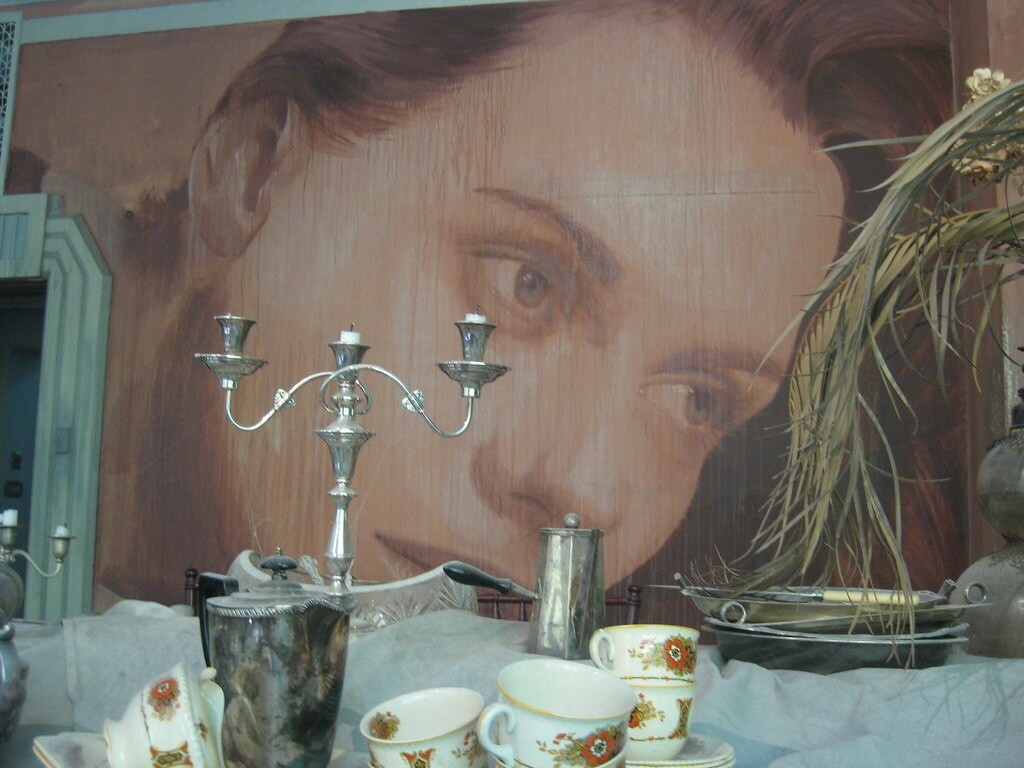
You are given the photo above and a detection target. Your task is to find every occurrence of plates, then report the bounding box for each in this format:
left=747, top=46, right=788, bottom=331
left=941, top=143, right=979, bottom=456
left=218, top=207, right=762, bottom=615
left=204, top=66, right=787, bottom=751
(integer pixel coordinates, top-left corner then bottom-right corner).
left=32, top=732, right=112, bottom=768
left=625, top=733, right=736, bottom=768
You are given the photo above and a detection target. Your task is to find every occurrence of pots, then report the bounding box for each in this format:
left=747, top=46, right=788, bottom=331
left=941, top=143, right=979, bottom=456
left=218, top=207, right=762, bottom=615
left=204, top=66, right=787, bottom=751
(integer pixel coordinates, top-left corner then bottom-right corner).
left=673, top=572, right=970, bottom=676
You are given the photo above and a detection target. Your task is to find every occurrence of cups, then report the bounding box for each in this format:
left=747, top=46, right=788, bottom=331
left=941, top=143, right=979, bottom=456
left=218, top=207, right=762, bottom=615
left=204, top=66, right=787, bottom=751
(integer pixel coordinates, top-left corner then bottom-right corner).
left=475, top=658, right=637, bottom=768
left=103, top=659, right=219, bottom=768
left=589, top=624, right=701, bottom=686
left=624, top=687, right=697, bottom=762
left=359, top=687, right=489, bottom=768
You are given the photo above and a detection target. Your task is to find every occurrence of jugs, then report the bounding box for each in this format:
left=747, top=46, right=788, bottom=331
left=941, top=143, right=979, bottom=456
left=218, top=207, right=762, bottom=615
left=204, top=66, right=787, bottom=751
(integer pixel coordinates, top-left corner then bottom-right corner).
left=443, top=513, right=607, bottom=660
left=196, top=548, right=358, bottom=768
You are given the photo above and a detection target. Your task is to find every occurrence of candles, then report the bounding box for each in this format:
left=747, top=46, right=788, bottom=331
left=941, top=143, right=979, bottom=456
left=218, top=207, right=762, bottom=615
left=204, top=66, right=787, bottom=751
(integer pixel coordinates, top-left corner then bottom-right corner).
left=56, top=523, right=70, bottom=536
left=341, top=321, right=361, bottom=345
left=467, top=304, right=487, bottom=324
left=3, top=507, right=18, bottom=526
